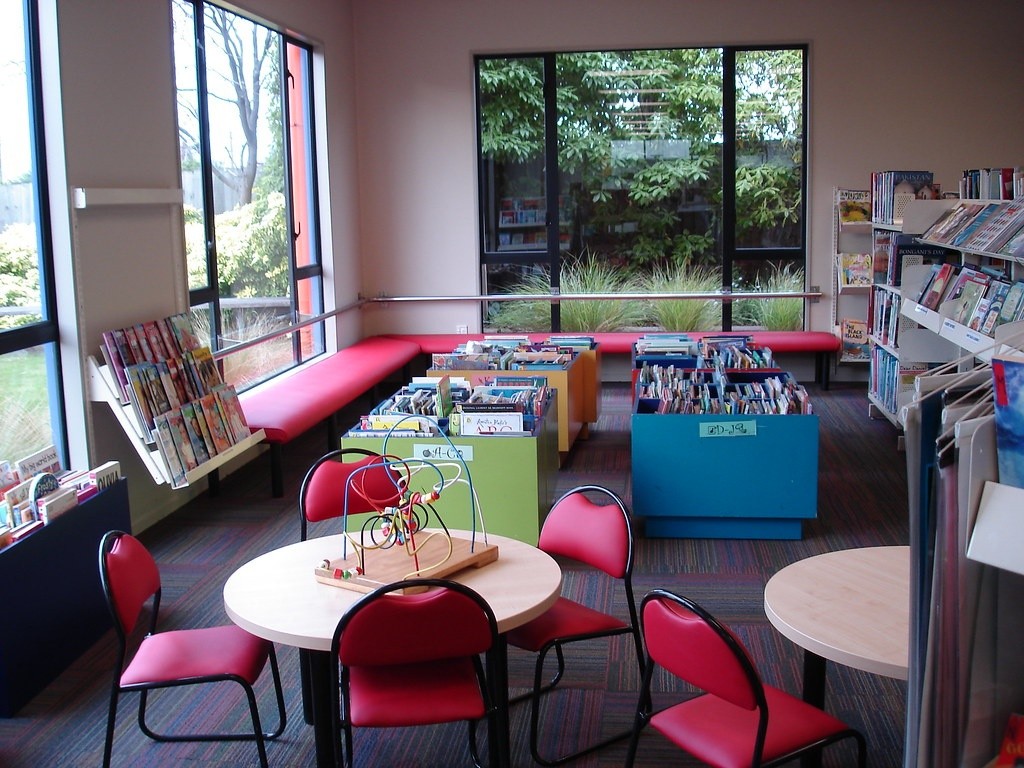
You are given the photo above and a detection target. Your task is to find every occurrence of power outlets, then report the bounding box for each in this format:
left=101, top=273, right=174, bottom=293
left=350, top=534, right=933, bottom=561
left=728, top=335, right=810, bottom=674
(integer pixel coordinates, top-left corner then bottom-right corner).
left=455, top=325, right=469, bottom=335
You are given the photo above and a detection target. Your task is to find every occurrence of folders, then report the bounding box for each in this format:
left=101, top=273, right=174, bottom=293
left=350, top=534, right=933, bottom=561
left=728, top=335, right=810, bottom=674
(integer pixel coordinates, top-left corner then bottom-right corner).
left=899, top=368, right=1024, bottom=768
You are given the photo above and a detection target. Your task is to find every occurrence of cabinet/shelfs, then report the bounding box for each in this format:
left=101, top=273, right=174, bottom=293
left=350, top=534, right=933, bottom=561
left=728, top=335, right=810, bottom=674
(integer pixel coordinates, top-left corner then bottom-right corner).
left=0, top=470, right=128, bottom=719
left=832, top=185, right=1024, bottom=451
left=632, top=342, right=820, bottom=540
left=498, top=183, right=802, bottom=292
left=341, top=342, right=601, bottom=548
left=70, top=184, right=267, bottom=490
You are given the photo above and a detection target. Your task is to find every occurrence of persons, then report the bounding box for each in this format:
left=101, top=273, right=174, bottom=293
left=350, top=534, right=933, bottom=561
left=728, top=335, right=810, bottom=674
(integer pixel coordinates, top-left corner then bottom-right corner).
left=200, top=361, right=221, bottom=393
left=223, top=394, right=249, bottom=443
left=150, top=335, right=168, bottom=363
left=208, top=405, right=231, bottom=451
left=190, top=418, right=210, bottom=460
left=179, top=327, right=200, bottom=354
left=177, top=419, right=198, bottom=471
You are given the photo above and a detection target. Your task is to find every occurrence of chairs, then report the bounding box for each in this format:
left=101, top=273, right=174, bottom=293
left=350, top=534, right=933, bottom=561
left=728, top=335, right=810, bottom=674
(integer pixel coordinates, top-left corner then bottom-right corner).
left=505, top=483, right=866, bottom=768
left=98, top=530, right=286, bottom=768
left=330, top=579, right=505, bottom=768
left=299, top=448, right=409, bottom=541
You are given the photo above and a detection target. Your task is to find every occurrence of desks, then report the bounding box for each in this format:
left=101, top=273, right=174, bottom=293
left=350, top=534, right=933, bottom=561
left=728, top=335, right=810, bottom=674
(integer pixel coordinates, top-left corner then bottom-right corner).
left=223, top=527, right=564, bottom=768
left=763, top=545, right=910, bottom=768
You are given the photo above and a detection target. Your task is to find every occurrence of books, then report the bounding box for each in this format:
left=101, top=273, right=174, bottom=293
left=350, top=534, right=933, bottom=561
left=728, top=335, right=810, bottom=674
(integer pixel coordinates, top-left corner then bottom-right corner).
left=494, top=193, right=571, bottom=252
left=833, top=166, right=1024, bottom=412
left=348, top=334, right=596, bottom=437
left=633, top=330, right=812, bottom=416
left=0, top=444, right=122, bottom=551
left=102, top=312, right=251, bottom=488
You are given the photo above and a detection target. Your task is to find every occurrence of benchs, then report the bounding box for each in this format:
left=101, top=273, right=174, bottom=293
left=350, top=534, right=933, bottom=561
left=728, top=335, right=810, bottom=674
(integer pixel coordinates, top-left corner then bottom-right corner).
left=231, top=331, right=841, bottom=498
